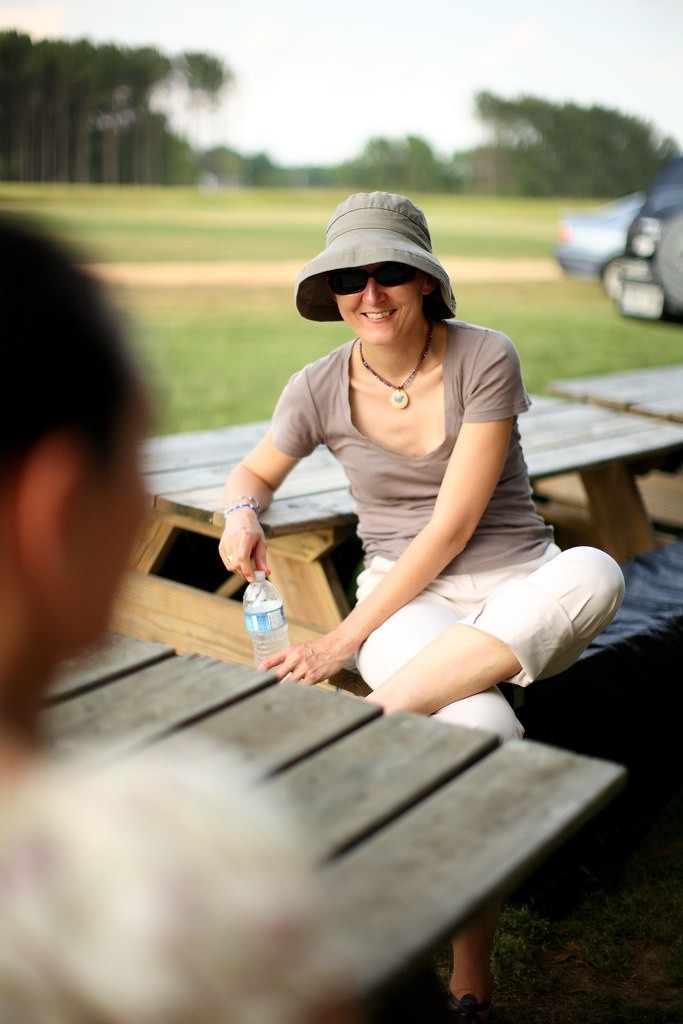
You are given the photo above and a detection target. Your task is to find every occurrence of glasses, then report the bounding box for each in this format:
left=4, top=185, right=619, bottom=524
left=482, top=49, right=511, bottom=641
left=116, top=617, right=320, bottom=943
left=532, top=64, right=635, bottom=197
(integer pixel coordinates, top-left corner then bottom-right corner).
left=329, top=260, right=415, bottom=296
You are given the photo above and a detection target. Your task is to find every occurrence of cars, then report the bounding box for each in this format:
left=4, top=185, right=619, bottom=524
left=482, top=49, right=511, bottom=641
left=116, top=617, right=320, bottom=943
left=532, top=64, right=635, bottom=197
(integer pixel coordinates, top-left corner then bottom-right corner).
left=553, top=159, right=683, bottom=318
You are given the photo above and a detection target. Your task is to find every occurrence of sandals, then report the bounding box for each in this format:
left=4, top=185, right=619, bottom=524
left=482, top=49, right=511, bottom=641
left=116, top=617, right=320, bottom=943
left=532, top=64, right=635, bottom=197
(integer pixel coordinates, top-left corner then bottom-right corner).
left=446, top=983, right=494, bottom=1024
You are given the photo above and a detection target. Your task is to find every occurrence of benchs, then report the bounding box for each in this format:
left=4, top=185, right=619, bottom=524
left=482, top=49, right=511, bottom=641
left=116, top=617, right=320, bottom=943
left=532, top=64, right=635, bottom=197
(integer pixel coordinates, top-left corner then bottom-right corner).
left=551, top=363, right=682, bottom=424
left=128, top=392, right=682, bottom=633
left=35, top=634, right=626, bottom=1024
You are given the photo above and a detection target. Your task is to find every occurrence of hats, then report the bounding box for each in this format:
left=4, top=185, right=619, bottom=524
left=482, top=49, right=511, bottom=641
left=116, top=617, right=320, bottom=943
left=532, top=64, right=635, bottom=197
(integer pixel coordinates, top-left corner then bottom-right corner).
left=294, top=192, right=455, bottom=322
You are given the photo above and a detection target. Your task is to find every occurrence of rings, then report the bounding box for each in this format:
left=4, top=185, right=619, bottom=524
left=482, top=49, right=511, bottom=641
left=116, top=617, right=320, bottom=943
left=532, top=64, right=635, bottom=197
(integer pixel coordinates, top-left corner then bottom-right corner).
left=225, top=554, right=234, bottom=563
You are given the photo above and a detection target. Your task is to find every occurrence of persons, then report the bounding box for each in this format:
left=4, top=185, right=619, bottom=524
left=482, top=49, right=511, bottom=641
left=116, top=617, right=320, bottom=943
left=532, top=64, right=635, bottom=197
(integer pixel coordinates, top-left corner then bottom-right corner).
left=3, top=191, right=374, bottom=1021
left=209, top=185, right=628, bottom=742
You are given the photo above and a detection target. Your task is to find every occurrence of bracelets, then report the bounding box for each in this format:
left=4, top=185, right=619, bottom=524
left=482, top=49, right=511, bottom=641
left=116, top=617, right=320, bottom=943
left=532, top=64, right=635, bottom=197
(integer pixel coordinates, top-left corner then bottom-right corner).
left=219, top=496, right=264, bottom=519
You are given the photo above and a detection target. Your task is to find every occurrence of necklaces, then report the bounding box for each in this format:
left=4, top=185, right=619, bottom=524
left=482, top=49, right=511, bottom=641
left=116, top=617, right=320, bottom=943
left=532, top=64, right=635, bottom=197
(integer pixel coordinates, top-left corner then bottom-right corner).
left=356, top=319, right=435, bottom=410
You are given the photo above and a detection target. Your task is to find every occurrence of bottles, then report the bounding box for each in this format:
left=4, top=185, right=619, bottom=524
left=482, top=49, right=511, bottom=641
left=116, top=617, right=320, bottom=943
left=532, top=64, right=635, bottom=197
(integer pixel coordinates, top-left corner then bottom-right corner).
left=243, top=570, right=290, bottom=682
